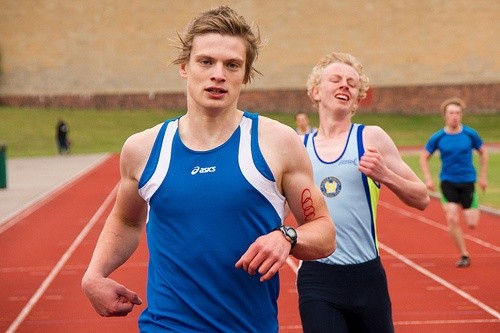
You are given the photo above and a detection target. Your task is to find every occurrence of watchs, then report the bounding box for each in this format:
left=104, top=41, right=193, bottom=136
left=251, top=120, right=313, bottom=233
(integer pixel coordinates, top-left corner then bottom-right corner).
left=273, top=224, right=297, bottom=254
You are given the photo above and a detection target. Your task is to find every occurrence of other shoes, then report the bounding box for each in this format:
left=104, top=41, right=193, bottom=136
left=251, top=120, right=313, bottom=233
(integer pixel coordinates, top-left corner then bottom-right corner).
left=456, top=254, right=470, bottom=267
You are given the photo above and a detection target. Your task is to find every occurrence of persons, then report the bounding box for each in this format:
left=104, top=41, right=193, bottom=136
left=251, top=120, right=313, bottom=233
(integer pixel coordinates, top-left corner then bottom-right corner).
left=294, top=112, right=318, bottom=135
left=296, top=51, right=430, bottom=333
left=55, top=116, right=71, bottom=155
left=420, top=97, right=487, bottom=267
left=81, top=6, right=337, bottom=333
